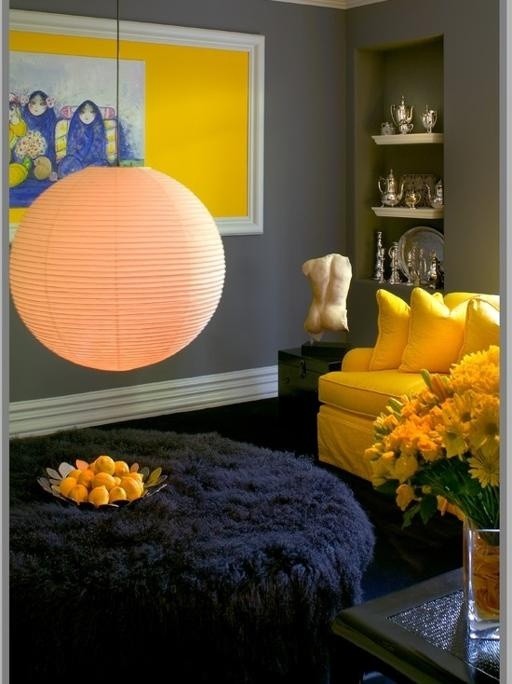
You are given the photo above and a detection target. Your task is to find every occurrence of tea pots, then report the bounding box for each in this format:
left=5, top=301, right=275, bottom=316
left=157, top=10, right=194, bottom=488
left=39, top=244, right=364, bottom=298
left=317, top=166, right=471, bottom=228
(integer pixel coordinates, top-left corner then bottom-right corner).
left=380, top=95, right=438, bottom=135
left=377, top=168, right=444, bottom=208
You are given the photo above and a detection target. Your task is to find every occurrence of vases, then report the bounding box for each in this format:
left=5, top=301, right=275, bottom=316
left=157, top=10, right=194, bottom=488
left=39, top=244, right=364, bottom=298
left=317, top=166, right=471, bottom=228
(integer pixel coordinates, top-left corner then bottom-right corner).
left=462, top=520, right=501, bottom=640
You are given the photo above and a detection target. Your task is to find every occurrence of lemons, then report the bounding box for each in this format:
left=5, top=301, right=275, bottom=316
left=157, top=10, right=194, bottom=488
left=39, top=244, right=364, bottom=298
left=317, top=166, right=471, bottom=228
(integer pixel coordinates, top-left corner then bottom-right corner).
left=59, top=455, right=144, bottom=505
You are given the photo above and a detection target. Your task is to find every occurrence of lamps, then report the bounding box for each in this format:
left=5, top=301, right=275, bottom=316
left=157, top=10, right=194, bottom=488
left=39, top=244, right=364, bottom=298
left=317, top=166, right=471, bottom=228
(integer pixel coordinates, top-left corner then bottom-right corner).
left=10, top=0, right=227, bottom=373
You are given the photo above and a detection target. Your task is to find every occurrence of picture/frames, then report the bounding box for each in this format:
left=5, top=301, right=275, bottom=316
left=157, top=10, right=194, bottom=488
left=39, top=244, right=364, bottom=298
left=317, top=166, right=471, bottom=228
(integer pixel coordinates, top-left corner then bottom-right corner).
left=8, top=8, right=267, bottom=245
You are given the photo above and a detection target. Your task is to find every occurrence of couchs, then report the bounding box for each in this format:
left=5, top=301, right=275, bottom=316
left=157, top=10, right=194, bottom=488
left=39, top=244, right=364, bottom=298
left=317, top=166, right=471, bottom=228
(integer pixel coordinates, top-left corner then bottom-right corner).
left=318, top=291, right=500, bottom=519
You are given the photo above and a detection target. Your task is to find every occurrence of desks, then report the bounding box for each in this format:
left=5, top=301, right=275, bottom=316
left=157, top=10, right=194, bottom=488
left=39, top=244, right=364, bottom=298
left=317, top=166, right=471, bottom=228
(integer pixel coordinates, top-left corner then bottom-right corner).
left=331, top=566, right=499, bottom=684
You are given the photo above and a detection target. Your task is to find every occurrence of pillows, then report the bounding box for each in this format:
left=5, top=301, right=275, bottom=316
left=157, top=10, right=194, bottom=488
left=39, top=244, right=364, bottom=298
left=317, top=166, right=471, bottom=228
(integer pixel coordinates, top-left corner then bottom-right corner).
left=368, top=287, right=499, bottom=371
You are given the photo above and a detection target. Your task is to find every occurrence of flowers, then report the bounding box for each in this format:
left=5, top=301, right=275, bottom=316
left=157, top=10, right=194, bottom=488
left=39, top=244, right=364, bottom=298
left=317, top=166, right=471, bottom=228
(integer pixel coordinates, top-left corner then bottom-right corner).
left=365, top=346, right=500, bottom=547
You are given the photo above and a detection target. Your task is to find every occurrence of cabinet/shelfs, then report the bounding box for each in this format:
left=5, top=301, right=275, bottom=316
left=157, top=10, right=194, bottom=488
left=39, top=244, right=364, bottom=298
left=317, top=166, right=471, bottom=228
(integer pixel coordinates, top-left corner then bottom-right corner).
left=371, top=133, right=444, bottom=220
left=278, top=349, right=348, bottom=474
